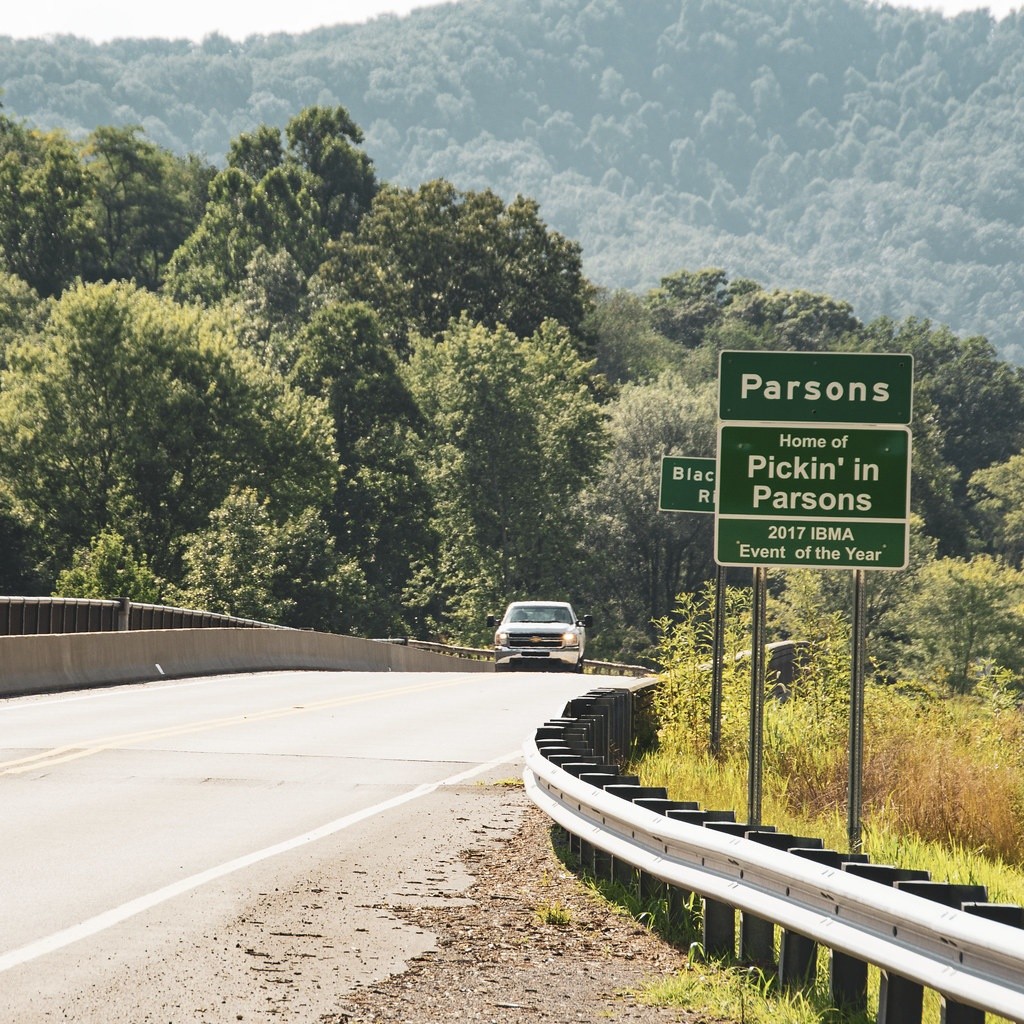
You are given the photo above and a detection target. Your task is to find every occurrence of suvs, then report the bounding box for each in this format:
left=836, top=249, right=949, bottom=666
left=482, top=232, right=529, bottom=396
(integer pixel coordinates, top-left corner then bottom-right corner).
left=485, top=600, right=593, bottom=674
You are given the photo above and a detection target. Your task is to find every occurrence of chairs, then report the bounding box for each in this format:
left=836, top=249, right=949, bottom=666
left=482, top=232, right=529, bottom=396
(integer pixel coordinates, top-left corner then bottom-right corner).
left=514, top=610, right=528, bottom=621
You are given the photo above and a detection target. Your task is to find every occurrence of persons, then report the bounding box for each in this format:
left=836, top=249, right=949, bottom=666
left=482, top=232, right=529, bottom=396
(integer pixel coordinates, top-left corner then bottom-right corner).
left=554, top=610, right=564, bottom=622
